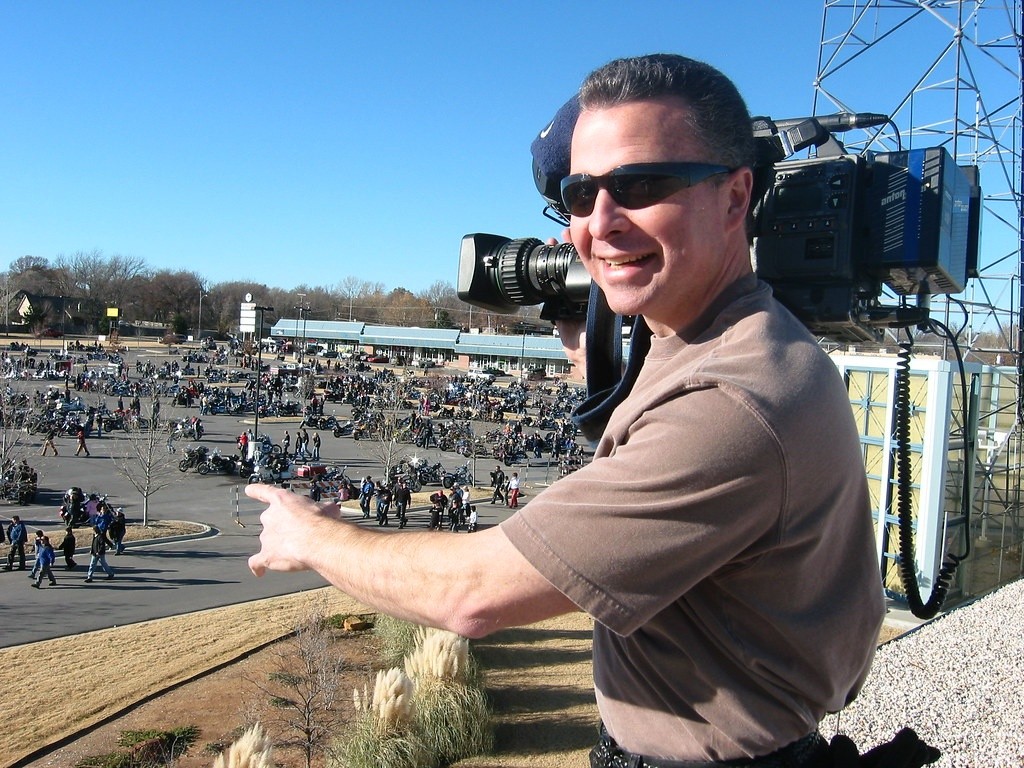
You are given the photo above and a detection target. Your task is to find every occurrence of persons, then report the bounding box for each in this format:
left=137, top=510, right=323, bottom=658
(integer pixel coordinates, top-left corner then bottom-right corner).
left=243, top=55, right=885, bottom=768
left=0, top=339, right=587, bottom=589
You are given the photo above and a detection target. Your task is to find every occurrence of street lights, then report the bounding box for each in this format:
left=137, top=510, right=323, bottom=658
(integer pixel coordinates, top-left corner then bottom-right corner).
left=517, top=320, right=529, bottom=382
left=197, top=290, right=209, bottom=338
left=301, top=307, right=312, bottom=363
left=250, top=305, right=275, bottom=441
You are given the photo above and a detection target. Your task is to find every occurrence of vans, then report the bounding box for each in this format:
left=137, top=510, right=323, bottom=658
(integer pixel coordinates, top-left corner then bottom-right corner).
left=525, top=368, right=547, bottom=380
left=391, top=356, right=407, bottom=365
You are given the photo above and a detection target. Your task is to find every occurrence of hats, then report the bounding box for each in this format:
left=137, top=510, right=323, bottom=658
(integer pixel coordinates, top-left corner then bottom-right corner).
left=117, top=507, right=123, bottom=513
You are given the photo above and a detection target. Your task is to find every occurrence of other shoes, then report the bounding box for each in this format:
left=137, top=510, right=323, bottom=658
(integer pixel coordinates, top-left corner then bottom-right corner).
left=120, top=544, right=126, bottom=552
left=115, top=552, right=120, bottom=556
left=31, top=584, right=39, bottom=589
left=107, top=572, right=114, bottom=579
left=84, top=578, right=92, bottom=583
left=49, top=581, right=56, bottom=586
left=27, top=575, right=35, bottom=580
left=16, top=567, right=26, bottom=570
left=66, top=563, right=76, bottom=570
left=2, top=566, right=12, bottom=571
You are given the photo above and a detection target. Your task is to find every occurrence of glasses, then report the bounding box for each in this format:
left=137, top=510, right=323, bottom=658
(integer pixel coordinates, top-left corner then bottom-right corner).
left=560, top=161, right=737, bottom=218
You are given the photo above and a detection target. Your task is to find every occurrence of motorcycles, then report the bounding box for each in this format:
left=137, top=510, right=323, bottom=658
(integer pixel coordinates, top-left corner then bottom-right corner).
left=101, top=407, right=148, bottom=431
left=403, top=422, right=511, bottom=464
left=390, top=457, right=474, bottom=489
left=27, top=411, right=82, bottom=435
left=179, top=446, right=239, bottom=474
left=299, top=409, right=386, bottom=440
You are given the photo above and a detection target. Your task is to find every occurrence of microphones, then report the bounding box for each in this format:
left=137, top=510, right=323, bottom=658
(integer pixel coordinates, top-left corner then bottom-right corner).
left=751, top=112, right=888, bottom=133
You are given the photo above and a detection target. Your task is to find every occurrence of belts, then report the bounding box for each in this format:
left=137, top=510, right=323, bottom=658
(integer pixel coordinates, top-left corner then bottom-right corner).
left=589, top=722, right=820, bottom=768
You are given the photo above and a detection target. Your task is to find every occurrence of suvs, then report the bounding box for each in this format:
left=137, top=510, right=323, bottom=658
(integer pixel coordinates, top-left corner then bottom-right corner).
left=164, top=334, right=187, bottom=345
left=469, top=368, right=497, bottom=385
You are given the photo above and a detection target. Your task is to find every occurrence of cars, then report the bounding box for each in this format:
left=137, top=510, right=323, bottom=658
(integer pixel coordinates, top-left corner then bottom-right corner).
left=412, top=357, right=435, bottom=368
left=353, top=351, right=387, bottom=363
left=321, top=349, right=337, bottom=357
